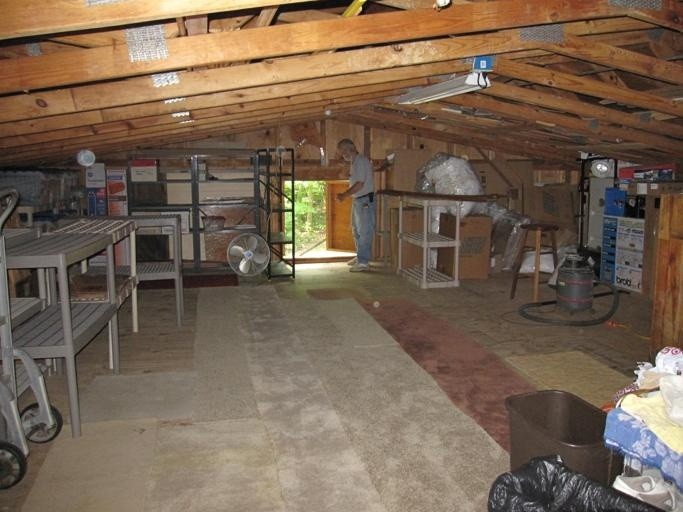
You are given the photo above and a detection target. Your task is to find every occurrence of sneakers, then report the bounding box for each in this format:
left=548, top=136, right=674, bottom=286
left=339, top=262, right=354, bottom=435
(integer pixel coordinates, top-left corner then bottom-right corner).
left=347, top=257, right=358, bottom=266
left=349, top=262, right=370, bottom=272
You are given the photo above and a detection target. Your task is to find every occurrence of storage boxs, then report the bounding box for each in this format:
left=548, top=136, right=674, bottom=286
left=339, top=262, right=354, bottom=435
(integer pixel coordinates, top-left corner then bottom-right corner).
left=438, top=208, right=492, bottom=281
left=599, top=188, right=646, bottom=296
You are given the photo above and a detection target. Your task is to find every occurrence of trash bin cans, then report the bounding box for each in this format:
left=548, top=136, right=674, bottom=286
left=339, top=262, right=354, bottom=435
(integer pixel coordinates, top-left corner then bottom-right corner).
left=493, top=389, right=669, bottom=512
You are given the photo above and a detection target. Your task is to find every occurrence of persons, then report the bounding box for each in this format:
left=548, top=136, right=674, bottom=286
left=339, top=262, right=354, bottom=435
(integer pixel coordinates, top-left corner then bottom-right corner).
left=333, top=137, right=376, bottom=272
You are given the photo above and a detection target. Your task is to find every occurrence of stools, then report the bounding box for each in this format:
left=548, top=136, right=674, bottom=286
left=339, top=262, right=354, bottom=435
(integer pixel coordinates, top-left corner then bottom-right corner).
left=510, top=219, right=560, bottom=301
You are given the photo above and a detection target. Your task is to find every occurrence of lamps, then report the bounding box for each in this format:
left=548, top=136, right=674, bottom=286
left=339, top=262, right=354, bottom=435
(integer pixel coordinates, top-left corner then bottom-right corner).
left=587, top=158, right=613, bottom=179
left=392, top=70, right=489, bottom=106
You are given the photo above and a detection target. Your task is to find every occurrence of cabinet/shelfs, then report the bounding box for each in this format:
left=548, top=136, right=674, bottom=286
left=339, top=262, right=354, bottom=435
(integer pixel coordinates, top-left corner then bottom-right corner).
left=0, top=201, right=187, bottom=460
left=124, top=146, right=297, bottom=280
left=396, top=189, right=460, bottom=288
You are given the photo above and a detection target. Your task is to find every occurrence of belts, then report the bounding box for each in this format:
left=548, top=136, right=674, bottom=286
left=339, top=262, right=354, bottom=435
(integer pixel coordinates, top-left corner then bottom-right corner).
left=355, top=192, right=374, bottom=199
